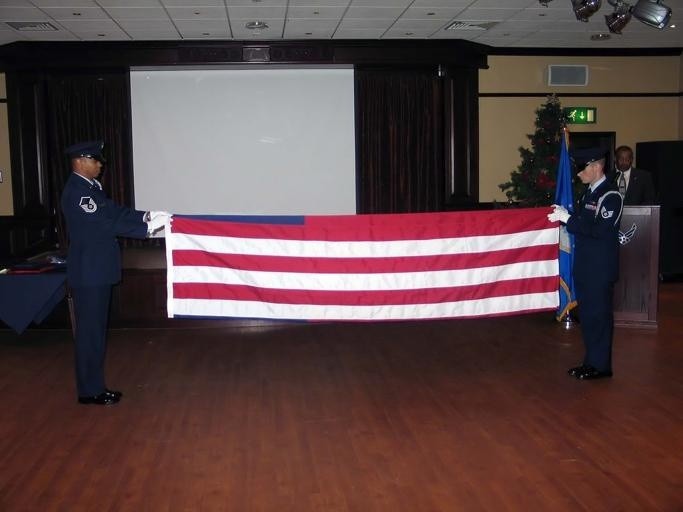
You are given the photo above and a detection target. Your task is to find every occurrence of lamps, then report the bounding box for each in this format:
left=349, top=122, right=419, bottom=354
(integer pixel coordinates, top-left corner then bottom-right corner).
left=569, top=0, right=633, bottom=35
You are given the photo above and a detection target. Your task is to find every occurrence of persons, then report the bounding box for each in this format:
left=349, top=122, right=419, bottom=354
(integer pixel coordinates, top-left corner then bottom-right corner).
left=547, top=146, right=624, bottom=379
left=59, top=139, right=172, bottom=403
left=605, top=145, right=656, bottom=206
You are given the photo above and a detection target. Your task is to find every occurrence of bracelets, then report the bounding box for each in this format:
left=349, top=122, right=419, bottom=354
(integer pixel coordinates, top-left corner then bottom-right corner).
left=147, top=210, right=151, bottom=221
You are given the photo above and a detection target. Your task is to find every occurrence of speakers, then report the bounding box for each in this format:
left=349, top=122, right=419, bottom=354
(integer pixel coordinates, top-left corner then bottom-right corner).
left=547, top=65, right=589, bottom=87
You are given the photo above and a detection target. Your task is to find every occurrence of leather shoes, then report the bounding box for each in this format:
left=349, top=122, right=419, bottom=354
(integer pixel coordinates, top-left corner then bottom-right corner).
left=79, top=390, right=123, bottom=404
left=567, top=364, right=613, bottom=380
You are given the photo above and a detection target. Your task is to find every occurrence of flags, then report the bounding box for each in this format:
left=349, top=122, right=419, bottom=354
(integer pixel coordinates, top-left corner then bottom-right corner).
left=555, top=130, right=578, bottom=323
left=167, top=209, right=563, bottom=326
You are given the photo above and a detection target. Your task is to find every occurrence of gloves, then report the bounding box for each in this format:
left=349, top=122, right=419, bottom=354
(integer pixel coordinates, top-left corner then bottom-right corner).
left=548, top=203, right=571, bottom=223
left=148, top=210, right=173, bottom=233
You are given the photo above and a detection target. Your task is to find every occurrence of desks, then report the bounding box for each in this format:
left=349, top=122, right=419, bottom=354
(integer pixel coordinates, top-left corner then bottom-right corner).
left=0, top=266, right=78, bottom=344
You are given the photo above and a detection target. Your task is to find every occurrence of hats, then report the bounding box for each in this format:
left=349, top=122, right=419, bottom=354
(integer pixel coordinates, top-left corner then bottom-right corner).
left=64, top=140, right=106, bottom=162
left=572, top=148, right=609, bottom=172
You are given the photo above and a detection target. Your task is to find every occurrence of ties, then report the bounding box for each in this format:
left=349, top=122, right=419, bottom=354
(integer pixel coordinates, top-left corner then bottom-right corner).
left=92, top=181, right=101, bottom=190
left=584, top=188, right=592, bottom=201
left=618, top=172, right=626, bottom=199
